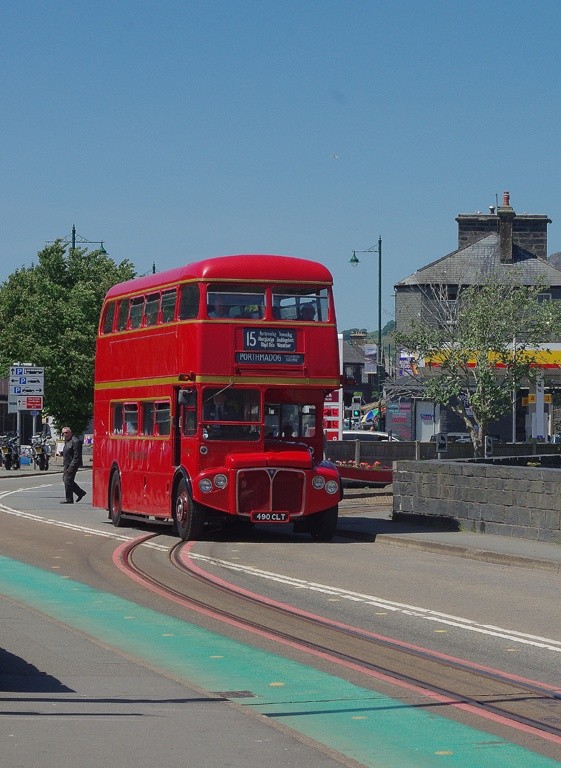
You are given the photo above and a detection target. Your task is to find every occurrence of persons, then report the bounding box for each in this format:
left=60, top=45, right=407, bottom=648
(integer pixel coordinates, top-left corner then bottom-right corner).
left=294, top=304, right=316, bottom=321
left=59, top=427, right=82, bottom=502
left=282, top=424, right=294, bottom=438
left=209, top=296, right=233, bottom=318
left=210, top=403, right=260, bottom=440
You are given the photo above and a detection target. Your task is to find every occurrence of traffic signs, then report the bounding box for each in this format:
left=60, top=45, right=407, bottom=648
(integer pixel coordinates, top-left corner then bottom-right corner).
left=10, top=367, right=45, bottom=376
left=16, top=396, right=44, bottom=411
left=10, top=376, right=44, bottom=385
left=8, top=385, right=45, bottom=395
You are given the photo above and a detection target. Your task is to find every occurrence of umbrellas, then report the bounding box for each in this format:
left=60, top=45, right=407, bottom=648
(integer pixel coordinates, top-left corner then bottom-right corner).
left=361, top=408, right=379, bottom=423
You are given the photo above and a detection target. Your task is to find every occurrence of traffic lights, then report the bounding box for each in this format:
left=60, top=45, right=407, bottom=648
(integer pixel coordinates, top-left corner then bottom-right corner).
left=351, top=396, right=361, bottom=419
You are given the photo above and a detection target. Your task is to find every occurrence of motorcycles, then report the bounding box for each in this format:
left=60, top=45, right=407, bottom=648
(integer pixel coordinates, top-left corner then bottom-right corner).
left=29, top=439, right=51, bottom=471
left=0, top=433, right=19, bottom=471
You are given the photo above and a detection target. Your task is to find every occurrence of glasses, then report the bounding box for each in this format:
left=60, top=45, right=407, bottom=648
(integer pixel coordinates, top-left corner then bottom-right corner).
left=63, top=430, right=70, bottom=435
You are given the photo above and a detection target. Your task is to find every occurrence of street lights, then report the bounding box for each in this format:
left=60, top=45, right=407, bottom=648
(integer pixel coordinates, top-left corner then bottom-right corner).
left=350, top=235, right=383, bottom=432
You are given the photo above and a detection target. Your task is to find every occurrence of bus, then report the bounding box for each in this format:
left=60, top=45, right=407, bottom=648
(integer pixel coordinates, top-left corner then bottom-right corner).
left=93, top=254, right=344, bottom=543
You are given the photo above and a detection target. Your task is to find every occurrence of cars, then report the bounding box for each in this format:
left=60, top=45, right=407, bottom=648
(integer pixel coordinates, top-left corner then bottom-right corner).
left=342, top=430, right=405, bottom=442
left=430, top=432, right=473, bottom=443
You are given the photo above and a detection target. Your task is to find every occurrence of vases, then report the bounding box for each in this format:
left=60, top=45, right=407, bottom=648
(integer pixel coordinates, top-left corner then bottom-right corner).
left=336, top=466, right=393, bottom=488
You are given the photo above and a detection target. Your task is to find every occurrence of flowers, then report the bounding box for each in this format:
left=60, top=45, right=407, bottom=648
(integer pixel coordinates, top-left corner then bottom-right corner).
left=327, top=457, right=392, bottom=470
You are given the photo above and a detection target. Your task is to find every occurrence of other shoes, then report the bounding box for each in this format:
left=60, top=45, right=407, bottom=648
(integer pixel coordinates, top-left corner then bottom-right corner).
left=60, top=500, right=74, bottom=504
left=76, top=491, right=87, bottom=503
